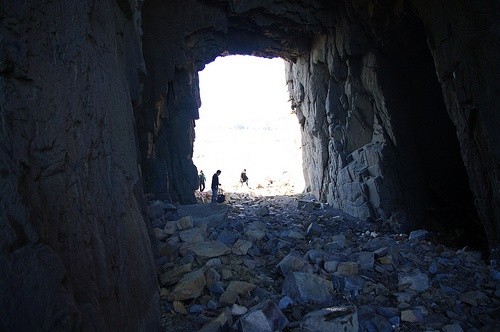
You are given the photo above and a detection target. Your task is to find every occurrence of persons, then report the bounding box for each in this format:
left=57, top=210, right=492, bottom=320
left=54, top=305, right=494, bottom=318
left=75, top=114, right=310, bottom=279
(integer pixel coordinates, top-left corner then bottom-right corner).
left=211, top=170, right=221, bottom=204
left=239, top=169, right=249, bottom=187
left=198, top=169, right=206, bottom=192
